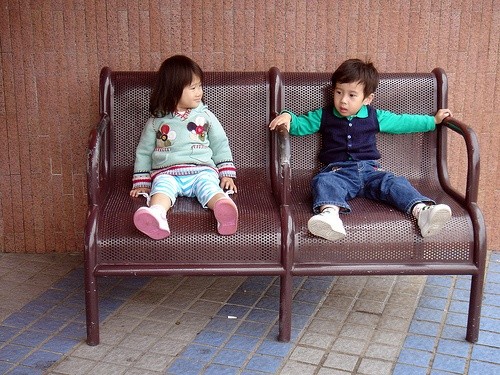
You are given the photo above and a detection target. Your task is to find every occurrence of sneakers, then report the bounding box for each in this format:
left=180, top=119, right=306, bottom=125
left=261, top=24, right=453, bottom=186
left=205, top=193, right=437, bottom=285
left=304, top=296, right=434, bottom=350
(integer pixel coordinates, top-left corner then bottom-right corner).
left=418, top=204, right=451, bottom=237
left=308, top=212, right=346, bottom=241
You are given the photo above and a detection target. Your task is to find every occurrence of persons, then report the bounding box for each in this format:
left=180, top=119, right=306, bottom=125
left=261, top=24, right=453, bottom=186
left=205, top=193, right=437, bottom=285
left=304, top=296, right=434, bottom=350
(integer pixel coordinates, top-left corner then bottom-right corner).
left=268, top=58, right=454, bottom=241
left=129, top=55, right=238, bottom=240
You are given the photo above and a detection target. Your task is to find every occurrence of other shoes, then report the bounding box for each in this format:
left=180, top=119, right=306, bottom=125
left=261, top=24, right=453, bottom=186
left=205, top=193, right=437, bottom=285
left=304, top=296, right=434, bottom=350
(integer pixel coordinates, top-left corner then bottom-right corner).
left=133, top=207, right=170, bottom=239
left=214, top=197, right=238, bottom=236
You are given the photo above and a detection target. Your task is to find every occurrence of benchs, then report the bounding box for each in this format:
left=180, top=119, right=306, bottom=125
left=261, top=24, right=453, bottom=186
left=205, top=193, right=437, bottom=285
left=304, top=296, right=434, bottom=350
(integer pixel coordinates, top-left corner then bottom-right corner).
left=82, top=66, right=487, bottom=346
